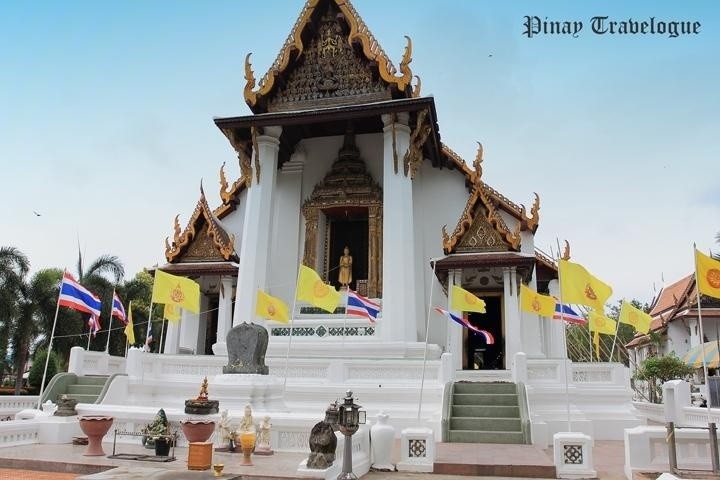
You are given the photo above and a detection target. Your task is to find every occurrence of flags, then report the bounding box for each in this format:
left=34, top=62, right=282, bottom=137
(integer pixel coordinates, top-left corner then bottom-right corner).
left=124, top=307, right=136, bottom=345
left=58, top=271, right=103, bottom=320
left=257, top=291, right=289, bottom=326
left=153, top=271, right=201, bottom=315
left=588, top=311, right=617, bottom=336
left=521, top=284, right=555, bottom=318
left=592, top=330, right=600, bottom=363
left=620, top=300, right=652, bottom=334
left=559, top=256, right=614, bottom=315
left=112, top=291, right=129, bottom=326
left=164, top=302, right=182, bottom=325
left=552, top=297, right=589, bottom=325
left=452, top=285, right=487, bottom=316
left=695, top=251, right=719, bottom=297
left=347, top=288, right=382, bottom=325
left=296, top=265, right=340, bottom=314
left=89, top=313, right=102, bottom=333
left=432, top=306, right=496, bottom=346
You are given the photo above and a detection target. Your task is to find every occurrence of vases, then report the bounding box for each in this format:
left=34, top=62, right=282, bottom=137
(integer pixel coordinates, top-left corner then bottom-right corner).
left=179, top=420, right=215, bottom=442
left=78, top=416, right=114, bottom=456
left=155, top=439, right=171, bottom=456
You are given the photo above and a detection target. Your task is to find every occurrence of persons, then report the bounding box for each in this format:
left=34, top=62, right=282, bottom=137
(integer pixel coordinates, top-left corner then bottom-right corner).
left=199, top=376, right=208, bottom=395
left=336, top=246, right=353, bottom=285
left=216, top=410, right=232, bottom=437
left=260, top=415, right=271, bottom=444
left=240, top=404, right=253, bottom=429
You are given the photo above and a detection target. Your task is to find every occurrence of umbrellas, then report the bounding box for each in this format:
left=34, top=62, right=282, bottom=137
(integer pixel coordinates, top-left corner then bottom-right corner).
left=677, top=338, right=720, bottom=369
